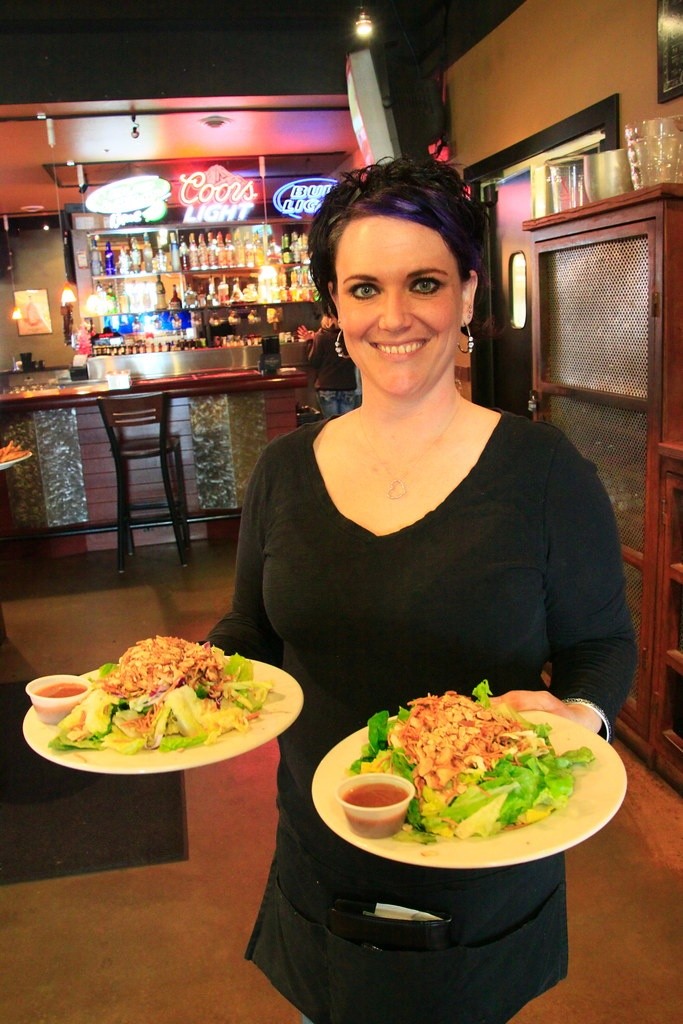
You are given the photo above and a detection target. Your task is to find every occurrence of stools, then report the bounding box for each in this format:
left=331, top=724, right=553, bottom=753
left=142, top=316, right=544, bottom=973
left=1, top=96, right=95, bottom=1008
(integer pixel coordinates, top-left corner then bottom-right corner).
left=96, top=391, right=192, bottom=575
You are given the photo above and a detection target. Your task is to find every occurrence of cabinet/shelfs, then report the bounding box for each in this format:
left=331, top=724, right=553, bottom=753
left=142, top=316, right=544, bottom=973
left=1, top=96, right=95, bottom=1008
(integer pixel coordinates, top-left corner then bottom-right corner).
left=523, top=184, right=683, bottom=798
left=83, top=217, right=321, bottom=357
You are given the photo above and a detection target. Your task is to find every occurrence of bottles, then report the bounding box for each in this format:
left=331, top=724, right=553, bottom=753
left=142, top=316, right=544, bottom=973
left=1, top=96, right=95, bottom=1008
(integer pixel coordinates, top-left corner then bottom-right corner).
left=88, top=227, right=321, bottom=338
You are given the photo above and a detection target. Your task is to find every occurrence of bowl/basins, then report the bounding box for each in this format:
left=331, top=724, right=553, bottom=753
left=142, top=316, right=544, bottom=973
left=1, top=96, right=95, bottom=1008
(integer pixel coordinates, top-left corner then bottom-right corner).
left=334, top=771, right=416, bottom=839
left=25, top=674, right=91, bottom=725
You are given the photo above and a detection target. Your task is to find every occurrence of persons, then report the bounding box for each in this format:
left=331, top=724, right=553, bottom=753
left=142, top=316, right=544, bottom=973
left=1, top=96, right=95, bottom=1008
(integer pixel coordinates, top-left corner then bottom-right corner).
left=200, top=156, right=638, bottom=1024
left=297, top=314, right=357, bottom=419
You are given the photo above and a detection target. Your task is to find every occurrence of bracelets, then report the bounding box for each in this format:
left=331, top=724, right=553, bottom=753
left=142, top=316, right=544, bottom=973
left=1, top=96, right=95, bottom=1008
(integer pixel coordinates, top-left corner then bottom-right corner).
left=563, top=697, right=610, bottom=745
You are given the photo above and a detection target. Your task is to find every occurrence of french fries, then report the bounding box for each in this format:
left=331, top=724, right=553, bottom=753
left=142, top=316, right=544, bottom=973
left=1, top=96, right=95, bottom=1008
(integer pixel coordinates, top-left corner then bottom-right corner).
left=0, top=440, right=29, bottom=463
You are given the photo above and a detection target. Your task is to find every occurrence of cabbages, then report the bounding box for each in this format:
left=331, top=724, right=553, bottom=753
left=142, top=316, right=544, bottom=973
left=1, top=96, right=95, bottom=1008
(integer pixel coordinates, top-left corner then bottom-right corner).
left=49, top=645, right=271, bottom=754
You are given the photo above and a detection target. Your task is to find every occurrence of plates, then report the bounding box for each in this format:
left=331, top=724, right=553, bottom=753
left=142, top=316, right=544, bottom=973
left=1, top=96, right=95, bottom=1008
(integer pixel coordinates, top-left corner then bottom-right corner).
left=22, top=655, right=303, bottom=775
left=0, top=452, right=32, bottom=470
left=312, top=710, right=628, bottom=870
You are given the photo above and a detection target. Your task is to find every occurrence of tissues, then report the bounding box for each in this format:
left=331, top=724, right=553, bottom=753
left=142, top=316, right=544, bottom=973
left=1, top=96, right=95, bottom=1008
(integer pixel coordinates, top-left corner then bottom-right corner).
left=69, top=354, right=89, bottom=382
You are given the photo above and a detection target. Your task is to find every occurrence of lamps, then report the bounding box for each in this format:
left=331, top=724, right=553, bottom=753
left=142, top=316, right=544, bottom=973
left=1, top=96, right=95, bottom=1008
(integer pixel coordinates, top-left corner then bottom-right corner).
left=129, top=112, right=140, bottom=139
left=75, top=163, right=103, bottom=314
left=355, top=8, right=375, bottom=36
left=43, top=118, right=77, bottom=306
left=3, top=216, right=24, bottom=320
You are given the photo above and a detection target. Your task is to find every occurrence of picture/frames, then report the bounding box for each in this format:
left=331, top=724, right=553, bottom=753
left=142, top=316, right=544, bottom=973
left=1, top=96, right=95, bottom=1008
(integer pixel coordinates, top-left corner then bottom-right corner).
left=11, top=288, right=54, bottom=337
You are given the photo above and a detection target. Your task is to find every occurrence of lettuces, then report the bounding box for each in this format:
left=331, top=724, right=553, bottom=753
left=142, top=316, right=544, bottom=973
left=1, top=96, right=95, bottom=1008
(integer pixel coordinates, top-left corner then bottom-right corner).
left=348, top=679, right=595, bottom=844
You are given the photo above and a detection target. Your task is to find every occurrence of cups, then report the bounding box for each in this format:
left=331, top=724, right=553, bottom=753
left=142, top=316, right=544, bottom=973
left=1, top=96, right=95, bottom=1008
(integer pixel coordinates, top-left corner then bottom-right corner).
left=20, top=352, right=33, bottom=372
left=545, top=115, right=683, bottom=215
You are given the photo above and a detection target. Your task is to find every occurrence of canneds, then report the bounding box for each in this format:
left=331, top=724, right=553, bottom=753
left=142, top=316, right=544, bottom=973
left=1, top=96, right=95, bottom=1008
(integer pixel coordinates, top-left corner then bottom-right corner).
left=92, top=343, right=146, bottom=356
